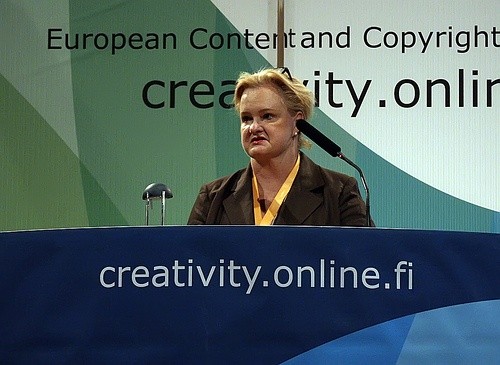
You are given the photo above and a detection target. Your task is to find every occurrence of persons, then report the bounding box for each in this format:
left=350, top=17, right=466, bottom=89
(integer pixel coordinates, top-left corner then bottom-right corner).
left=185, top=69, right=377, bottom=228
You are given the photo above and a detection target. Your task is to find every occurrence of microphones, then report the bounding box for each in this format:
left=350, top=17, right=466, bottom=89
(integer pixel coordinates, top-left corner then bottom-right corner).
left=296, top=119, right=370, bottom=227
